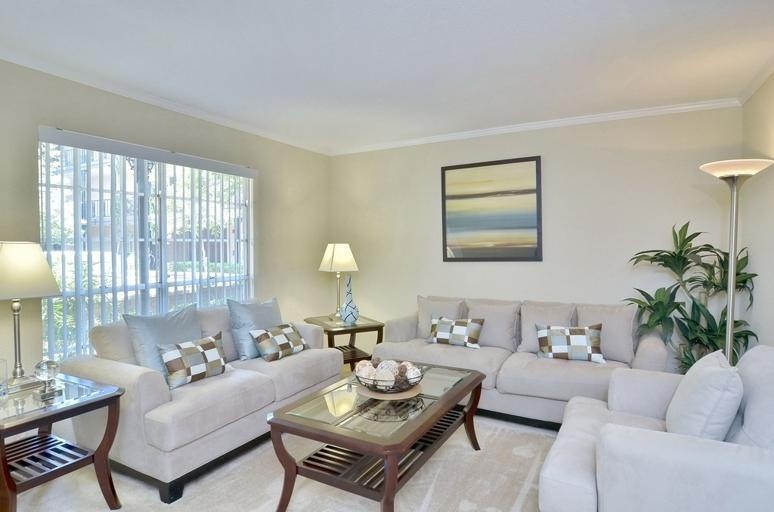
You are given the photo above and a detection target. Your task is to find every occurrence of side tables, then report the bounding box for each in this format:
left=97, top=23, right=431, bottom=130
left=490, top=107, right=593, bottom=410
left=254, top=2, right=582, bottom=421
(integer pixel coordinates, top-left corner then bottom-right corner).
left=304, top=314, right=385, bottom=371
left=0, top=374, right=125, bottom=512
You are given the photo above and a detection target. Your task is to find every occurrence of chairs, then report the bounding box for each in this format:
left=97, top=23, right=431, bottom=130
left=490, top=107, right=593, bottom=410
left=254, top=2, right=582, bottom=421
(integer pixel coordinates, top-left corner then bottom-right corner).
left=537, top=343, right=774, bottom=511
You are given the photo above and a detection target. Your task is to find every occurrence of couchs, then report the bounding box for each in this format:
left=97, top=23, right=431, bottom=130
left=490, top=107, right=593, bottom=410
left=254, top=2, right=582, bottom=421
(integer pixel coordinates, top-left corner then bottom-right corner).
left=62, top=296, right=344, bottom=503
left=372, top=295, right=668, bottom=432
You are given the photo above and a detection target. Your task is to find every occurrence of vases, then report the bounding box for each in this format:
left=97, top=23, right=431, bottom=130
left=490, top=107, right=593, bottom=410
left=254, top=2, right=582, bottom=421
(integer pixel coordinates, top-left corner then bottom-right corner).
left=340, top=274, right=360, bottom=323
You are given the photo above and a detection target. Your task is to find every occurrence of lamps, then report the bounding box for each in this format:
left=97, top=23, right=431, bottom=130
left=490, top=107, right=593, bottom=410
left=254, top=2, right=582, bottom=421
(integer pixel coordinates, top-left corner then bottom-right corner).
left=0, top=241, right=61, bottom=394
left=324, top=385, right=357, bottom=417
left=318, top=243, right=359, bottom=321
left=699, top=160, right=774, bottom=365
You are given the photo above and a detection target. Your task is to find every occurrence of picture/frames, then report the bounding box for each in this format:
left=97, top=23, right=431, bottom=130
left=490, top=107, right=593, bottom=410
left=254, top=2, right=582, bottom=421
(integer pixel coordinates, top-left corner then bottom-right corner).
left=441, top=155, right=543, bottom=262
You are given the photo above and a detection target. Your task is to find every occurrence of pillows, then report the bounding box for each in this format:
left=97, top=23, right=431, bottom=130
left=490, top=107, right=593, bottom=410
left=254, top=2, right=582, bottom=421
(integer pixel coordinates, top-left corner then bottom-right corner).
left=665, top=348, right=744, bottom=441
left=123, top=296, right=307, bottom=391
left=416, top=295, right=639, bottom=365
